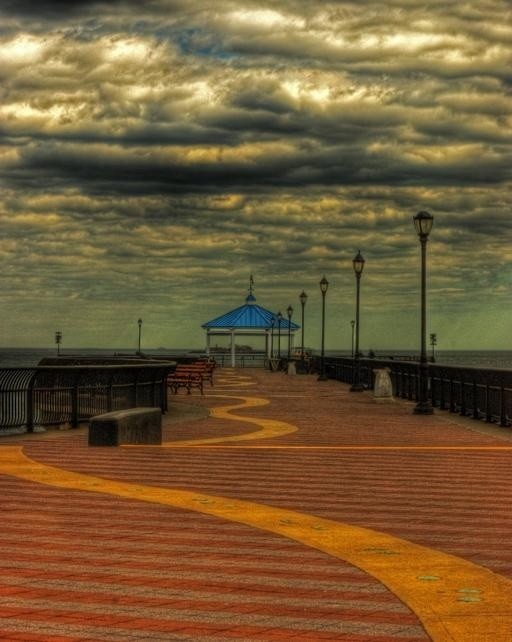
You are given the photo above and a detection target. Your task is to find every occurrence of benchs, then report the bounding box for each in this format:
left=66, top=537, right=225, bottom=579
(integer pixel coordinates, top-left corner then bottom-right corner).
left=164, top=362, right=217, bottom=395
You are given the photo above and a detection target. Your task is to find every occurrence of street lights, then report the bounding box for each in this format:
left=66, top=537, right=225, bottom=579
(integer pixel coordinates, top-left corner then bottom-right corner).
left=53, top=329, right=64, bottom=356
left=138, top=318, right=142, bottom=353
left=350, top=249, right=364, bottom=356
left=430, top=332, right=437, bottom=356
left=411, top=211, right=433, bottom=365
left=270, top=274, right=330, bottom=358
